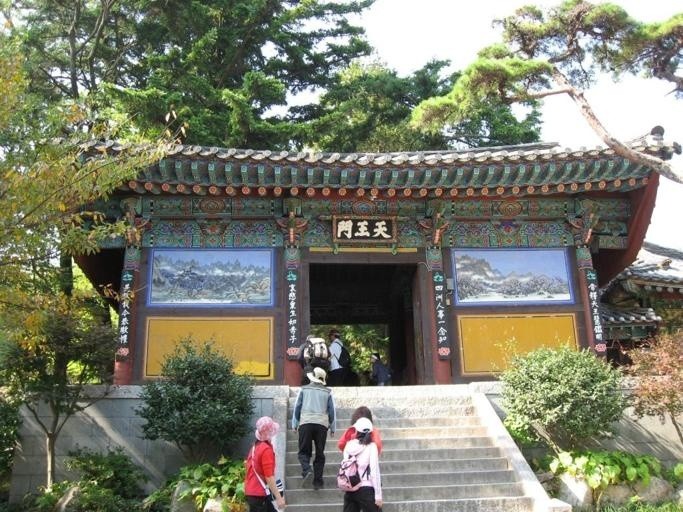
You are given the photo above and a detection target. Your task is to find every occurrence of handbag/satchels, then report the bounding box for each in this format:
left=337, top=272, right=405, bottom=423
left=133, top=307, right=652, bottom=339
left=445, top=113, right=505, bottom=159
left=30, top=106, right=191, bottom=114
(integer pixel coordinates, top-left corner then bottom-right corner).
left=263, top=478, right=286, bottom=512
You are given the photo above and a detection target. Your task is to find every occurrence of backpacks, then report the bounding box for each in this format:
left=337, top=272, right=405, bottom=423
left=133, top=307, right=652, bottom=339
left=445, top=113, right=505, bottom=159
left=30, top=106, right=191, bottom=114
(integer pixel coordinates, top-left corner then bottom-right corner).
left=334, top=342, right=351, bottom=367
left=304, top=337, right=331, bottom=367
left=336, top=449, right=369, bottom=491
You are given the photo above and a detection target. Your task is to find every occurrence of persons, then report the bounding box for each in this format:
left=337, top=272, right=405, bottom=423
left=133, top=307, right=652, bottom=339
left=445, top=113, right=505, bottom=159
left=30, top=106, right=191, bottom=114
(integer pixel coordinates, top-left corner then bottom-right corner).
left=244, top=415, right=286, bottom=512
left=328, top=330, right=353, bottom=386
left=296, top=335, right=330, bottom=386
left=337, top=416, right=382, bottom=512
left=337, top=405, right=382, bottom=459
left=291, top=367, right=336, bottom=489
left=368, top=353, right=393, bottom=386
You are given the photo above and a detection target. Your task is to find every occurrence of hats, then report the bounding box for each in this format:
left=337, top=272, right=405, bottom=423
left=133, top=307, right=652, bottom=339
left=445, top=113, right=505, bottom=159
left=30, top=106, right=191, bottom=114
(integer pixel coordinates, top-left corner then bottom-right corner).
left=254, top=416, right=279, bottom=441
left=372, top=353, right=380, bottom=361
left=306, top=367, right=328, bottom=386
left=348, top=417, right=372, bottom=434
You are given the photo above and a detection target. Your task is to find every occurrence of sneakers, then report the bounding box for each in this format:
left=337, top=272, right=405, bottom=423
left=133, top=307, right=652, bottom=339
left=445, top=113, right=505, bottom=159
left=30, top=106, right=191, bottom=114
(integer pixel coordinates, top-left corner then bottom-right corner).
left=300, top=470, right=324, bottom=491
